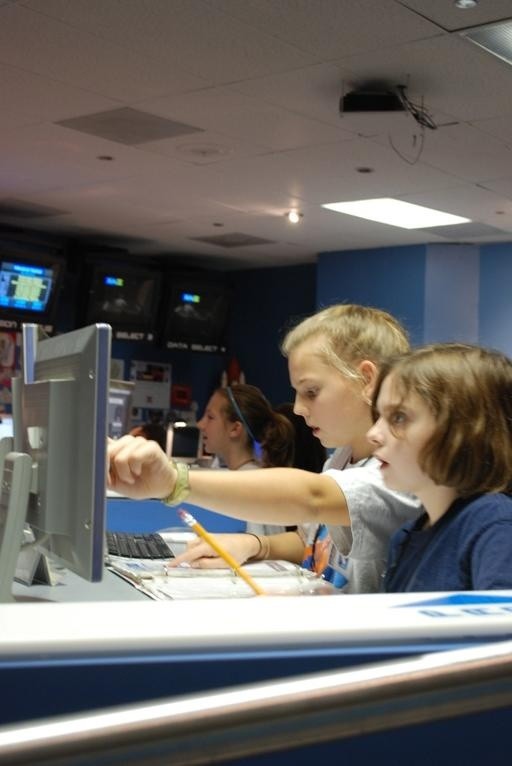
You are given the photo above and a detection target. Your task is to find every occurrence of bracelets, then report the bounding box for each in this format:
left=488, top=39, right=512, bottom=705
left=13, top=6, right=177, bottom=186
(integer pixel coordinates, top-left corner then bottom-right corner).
left=239, top=529, right=272, bottom=564
left=164, top=459, right=194, bottom=508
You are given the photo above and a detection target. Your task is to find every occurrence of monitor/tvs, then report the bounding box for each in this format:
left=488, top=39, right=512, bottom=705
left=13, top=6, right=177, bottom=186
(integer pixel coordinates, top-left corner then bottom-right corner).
left=20, top=322, right=52, bottom=384
left=160, top=271, right=234, bottom=347
left=78, top=252, right=162, bottom=331
left=0, top=322, right=115, bottom=607
left=107, top=380, right=136, bottom=440
left=0, top=247, right=61, bottom=325
left=165, top=424, right=204, bottom=464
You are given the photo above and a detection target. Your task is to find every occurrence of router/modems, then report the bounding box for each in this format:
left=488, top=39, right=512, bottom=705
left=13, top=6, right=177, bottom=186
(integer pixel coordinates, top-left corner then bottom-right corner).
left=334, top=90, right=426, bottom=136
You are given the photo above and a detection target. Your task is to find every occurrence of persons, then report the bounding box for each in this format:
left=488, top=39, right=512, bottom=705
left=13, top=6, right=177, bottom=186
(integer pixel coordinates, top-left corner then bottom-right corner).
left=272, top=400, right=327, bottom=479
left=124, top=423, right=166, bottom=453
left=196, top=380, right=298, bottom=540
left=101, top=304, right=429, bottom=596
left=362, top=340, right=512, bottom=592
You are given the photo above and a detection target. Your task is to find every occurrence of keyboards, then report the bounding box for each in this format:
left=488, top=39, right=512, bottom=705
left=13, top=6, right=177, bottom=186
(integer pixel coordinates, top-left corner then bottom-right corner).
left=106, top=529, right=176, bottom=558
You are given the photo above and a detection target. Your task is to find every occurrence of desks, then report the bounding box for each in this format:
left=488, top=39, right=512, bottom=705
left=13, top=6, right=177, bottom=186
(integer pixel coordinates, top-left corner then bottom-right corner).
left=96, top=497, right=247, bottom=538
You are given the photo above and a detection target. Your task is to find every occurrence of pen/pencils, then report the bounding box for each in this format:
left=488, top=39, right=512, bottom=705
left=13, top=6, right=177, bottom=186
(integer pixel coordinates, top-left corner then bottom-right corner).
left=177, top=508, right=269, bottom=596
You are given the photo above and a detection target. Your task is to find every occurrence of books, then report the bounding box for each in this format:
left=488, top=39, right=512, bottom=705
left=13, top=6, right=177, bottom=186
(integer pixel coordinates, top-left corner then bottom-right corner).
left=105, top=558, right=339, bottom=600
